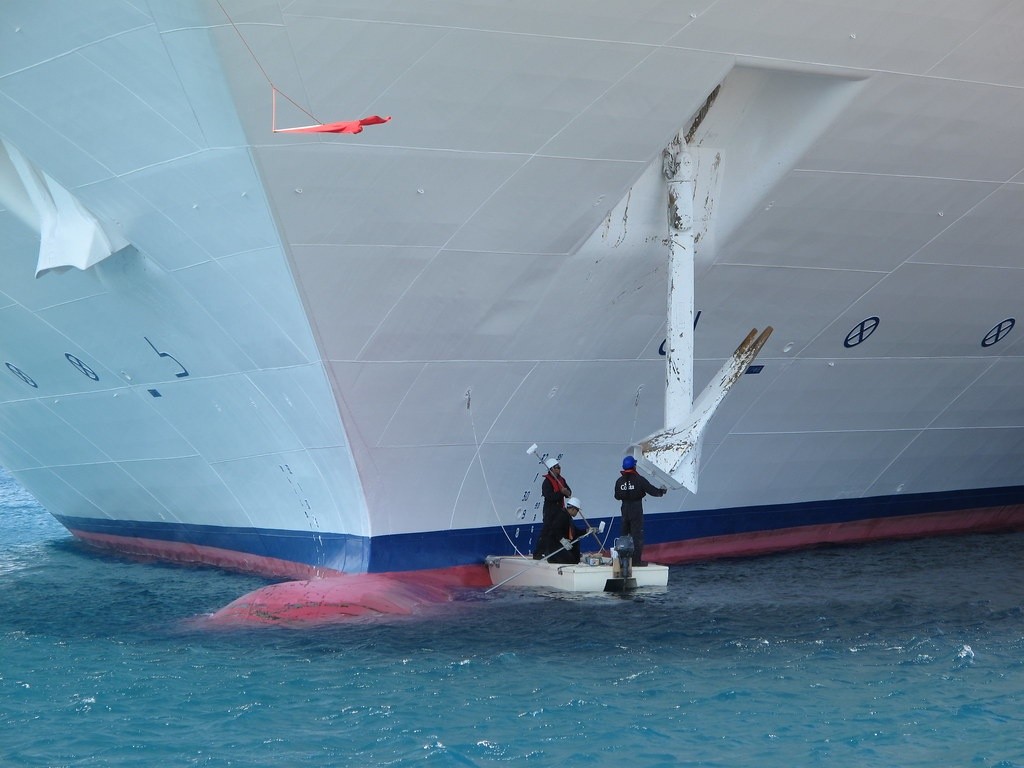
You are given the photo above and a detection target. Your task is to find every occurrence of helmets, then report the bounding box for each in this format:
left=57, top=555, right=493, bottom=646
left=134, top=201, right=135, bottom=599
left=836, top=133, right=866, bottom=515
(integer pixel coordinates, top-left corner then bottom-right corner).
left=567, top=497, right=583, bottom=510
left=546, top=458, right=559, bottom=471
left=623, top=456, right=637, bottom=470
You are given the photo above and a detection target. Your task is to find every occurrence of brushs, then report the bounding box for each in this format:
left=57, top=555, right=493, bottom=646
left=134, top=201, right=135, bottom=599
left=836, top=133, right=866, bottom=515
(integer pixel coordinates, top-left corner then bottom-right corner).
left=484, top=521, right=606, bottom=593
left=526, top=443, right=609, bottom=554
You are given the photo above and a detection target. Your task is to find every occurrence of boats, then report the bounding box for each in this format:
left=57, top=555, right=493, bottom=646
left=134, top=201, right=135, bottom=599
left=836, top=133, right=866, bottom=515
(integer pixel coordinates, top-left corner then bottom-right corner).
left=483, top=552, right=671, bottom=596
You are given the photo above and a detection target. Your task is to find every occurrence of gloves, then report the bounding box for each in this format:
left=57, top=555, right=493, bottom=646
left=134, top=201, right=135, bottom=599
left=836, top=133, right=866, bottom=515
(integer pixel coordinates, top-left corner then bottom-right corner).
left=586, top=527, right=599, bottom=534
left=660, top=485, right=667, bottom=495
left=560, top=537, right=573, bottom=550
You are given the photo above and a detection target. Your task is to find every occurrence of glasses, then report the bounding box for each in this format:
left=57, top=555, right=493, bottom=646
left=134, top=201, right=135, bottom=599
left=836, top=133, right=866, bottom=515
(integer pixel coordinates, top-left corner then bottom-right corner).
left=553, top=466, right=561, bottom=470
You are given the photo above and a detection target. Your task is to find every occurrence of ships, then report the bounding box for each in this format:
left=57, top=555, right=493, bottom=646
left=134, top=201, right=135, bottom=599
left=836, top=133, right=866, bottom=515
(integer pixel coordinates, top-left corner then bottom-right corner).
left=2, top=0, right=1024, bottom=631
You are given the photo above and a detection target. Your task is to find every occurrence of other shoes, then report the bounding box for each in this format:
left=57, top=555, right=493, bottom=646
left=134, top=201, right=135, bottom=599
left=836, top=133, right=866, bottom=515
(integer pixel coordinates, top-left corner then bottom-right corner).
left=633, top=561, right=648, bottom=567
left=580, top=552, right=583, bottom=557
left=532, top=554, right=545, bottom=560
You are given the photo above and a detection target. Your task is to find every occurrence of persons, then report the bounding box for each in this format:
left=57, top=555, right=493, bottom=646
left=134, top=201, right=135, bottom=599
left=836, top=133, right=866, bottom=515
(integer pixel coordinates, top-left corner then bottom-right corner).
left=533, top=458, right=572, bottom=561
left=541, top=497, right=600, bottom=565
left=614, top=456, right=668, bottom=567
